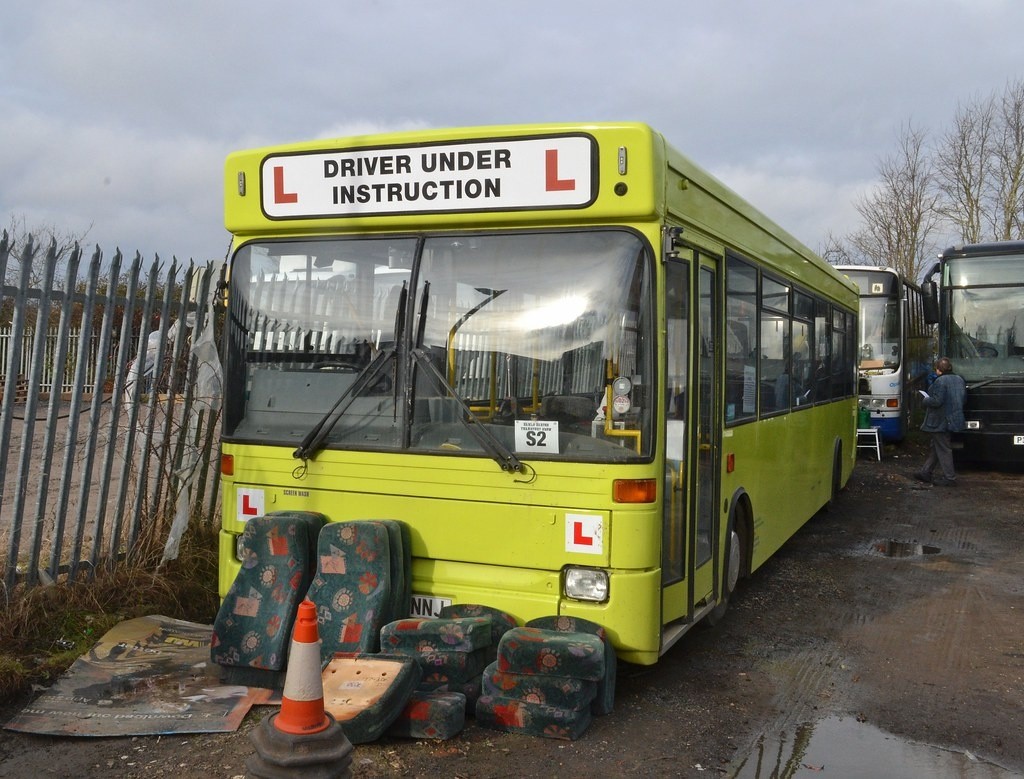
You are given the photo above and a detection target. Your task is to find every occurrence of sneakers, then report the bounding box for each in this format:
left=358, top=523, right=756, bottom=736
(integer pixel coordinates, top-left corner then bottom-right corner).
left=933, top=475, right=957, bottom=487
left=913, top=472, right=931, bottom=483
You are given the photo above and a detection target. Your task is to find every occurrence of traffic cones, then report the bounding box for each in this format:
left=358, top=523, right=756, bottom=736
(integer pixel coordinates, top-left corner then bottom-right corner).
left=247, top=595, right=354, bottom=779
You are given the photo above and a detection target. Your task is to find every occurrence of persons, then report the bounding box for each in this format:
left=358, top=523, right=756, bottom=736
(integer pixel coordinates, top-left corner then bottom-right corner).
left=773, top=351, right=804, bottom=410
left=912, top=356, right=966, bottom=487
left=866, top=322, right=890, bottom=343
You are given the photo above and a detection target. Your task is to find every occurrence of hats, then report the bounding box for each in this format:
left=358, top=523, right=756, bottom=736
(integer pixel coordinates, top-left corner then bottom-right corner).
left=938, top=357, right=952, bottom=370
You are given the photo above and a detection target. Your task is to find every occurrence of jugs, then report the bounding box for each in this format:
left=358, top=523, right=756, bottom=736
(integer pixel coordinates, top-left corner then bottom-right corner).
left=857, top=406, right=872, bottom=429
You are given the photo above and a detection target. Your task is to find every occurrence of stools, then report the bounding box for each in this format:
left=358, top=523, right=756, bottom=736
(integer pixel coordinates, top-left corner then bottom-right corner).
left=857, top=426, right=884, bottom=460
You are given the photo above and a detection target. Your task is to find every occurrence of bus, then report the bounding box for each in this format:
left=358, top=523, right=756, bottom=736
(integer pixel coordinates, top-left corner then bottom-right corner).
left=782, top=265, right=934, bottom=448
left=217, top=120, right=864, bottom=668
left=919, top=237, right=1024, bottom=470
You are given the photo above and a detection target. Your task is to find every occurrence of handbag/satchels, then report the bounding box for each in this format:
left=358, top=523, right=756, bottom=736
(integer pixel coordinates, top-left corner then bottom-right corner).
left=858, top=406, right=870, bottom=428
